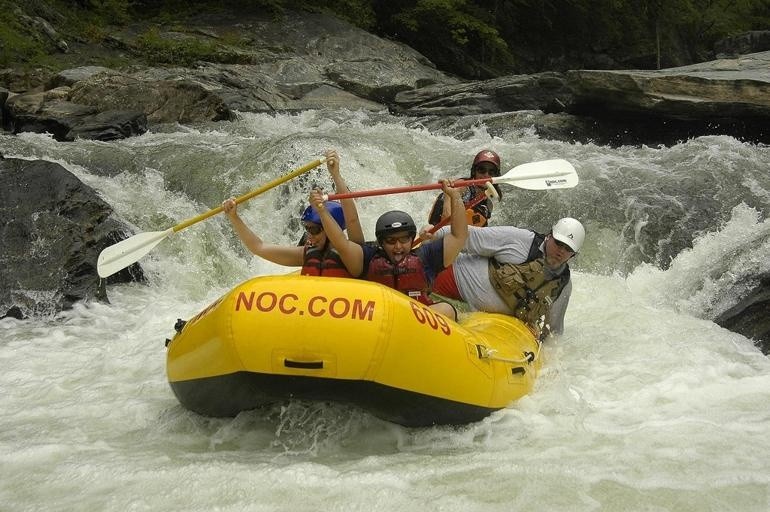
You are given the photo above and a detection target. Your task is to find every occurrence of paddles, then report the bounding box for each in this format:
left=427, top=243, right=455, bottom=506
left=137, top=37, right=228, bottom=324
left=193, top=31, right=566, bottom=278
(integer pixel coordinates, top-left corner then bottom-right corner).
left=97, top=156, right=336, bottom=279
left=321, top=159, right=578, bottom=202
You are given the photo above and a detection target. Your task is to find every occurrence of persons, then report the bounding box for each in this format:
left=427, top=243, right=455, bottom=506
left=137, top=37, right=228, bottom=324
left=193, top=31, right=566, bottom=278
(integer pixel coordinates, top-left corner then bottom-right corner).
left=310, top=177, right=469, bottom=322
left=422, top=208, right=587, bottom=339
left=428, top=150, right=502, bottom=228
left=222, top=150, right=367, bottom=280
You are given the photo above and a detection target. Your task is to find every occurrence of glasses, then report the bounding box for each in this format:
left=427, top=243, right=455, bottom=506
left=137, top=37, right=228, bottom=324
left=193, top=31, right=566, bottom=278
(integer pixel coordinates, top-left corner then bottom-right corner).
left=553, top=238, right=574, bottom=253
left=385, top=236, right=410, bottom=244
left=304, top=225, right=322, bottom=235
left=478, top=166, right=498, bottom=175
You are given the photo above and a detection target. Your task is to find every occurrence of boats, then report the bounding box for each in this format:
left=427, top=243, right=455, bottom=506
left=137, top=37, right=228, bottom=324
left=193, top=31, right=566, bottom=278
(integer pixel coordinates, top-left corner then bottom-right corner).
left=164, top=266, right=549, bottom=430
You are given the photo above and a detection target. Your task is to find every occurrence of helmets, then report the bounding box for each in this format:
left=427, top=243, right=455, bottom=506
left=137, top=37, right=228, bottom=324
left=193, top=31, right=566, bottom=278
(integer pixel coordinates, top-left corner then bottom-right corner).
left=301, top=201, right=346, bottom=232
left=374, top=212, right=417, bottom=235
left=474, top=150, right=500, bottom=172
left=551, top=217, right=586, bottom=254
